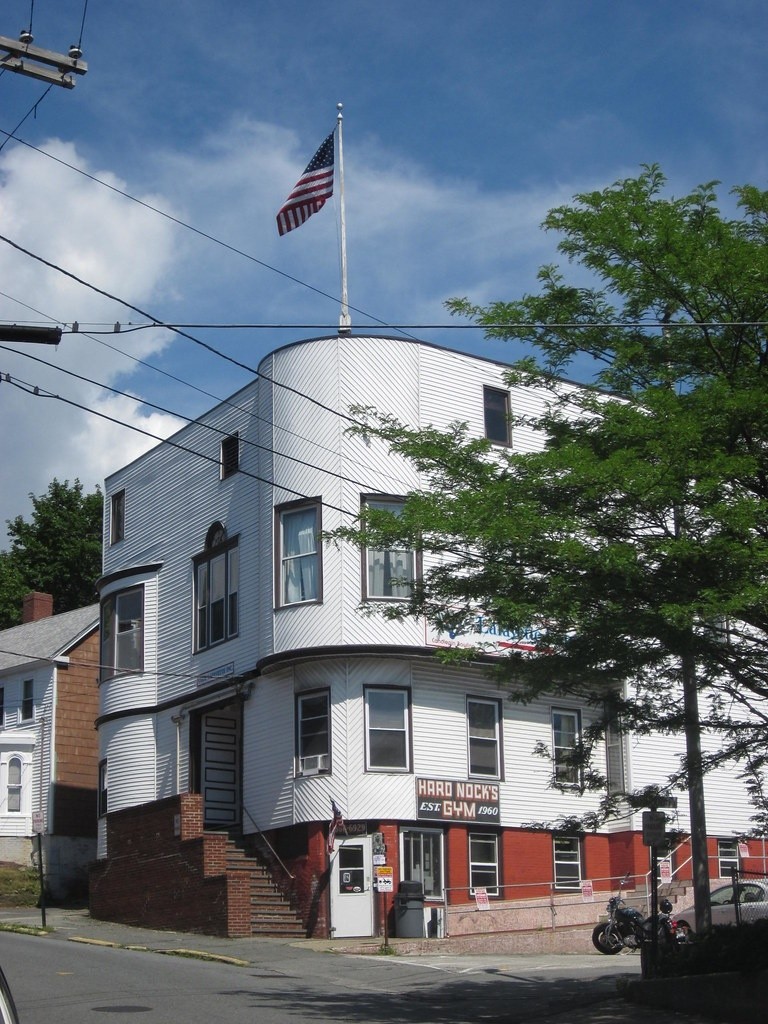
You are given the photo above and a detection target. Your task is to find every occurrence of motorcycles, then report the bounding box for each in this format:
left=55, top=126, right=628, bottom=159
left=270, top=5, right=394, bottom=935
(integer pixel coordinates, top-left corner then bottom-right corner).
left=590, top=872, right=678, bottom=956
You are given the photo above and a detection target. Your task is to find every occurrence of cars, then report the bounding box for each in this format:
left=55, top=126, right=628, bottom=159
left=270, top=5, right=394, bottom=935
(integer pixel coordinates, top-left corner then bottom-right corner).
left=673, top=880, right=768, bottom=940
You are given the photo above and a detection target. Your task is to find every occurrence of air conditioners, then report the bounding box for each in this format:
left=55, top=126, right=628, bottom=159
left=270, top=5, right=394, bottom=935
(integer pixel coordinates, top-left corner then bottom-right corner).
left=303, top=754, right=328, bottom=776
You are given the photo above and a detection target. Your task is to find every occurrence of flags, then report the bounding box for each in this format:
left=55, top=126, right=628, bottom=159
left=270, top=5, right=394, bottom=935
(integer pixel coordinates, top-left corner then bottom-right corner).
left=326, top=800, right=344, bottom=855
left=276, top=131, right=335, bottom=236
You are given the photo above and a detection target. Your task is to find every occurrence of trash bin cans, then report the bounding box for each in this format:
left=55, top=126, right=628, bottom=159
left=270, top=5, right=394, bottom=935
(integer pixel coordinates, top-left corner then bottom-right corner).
left=393, top=879, right=425, bottom=938
